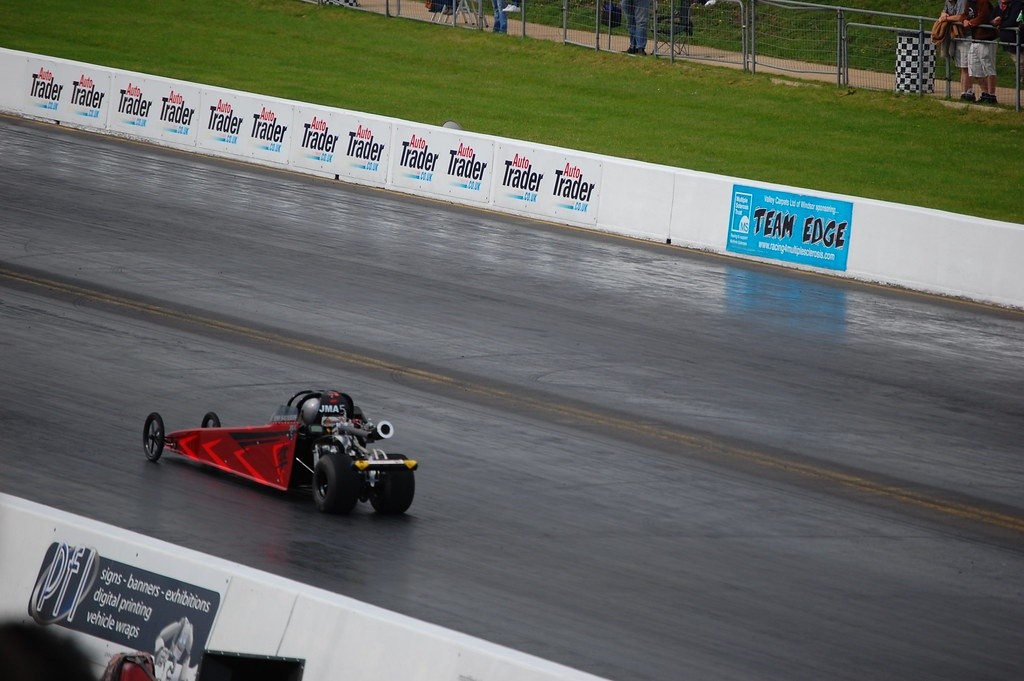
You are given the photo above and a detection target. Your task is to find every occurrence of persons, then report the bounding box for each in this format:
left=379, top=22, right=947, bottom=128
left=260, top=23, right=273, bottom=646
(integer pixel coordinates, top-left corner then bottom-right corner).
left=993, top=0, right=1023, bottom=87
left=620, top=1, right=650, bottom=56
left=942, top=0, right=975, bottom=100
left=490, top=0, right=508, bottom=34
left=963, top=0, right=998, bottom=105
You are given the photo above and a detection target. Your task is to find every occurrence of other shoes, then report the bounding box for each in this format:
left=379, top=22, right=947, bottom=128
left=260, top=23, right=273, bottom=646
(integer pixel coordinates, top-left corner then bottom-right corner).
left=501, top=4, right=521, bottom=13
left=624, top=46, right=647, bottom=56
left=960, top=92, right=975, bottom=102
left=977, top=92, right=997, bottom=104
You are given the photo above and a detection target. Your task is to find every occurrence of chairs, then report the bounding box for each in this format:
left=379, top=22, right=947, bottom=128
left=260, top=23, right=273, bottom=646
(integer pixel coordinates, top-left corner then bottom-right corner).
left=649, top=15, right=691, bottom=57
